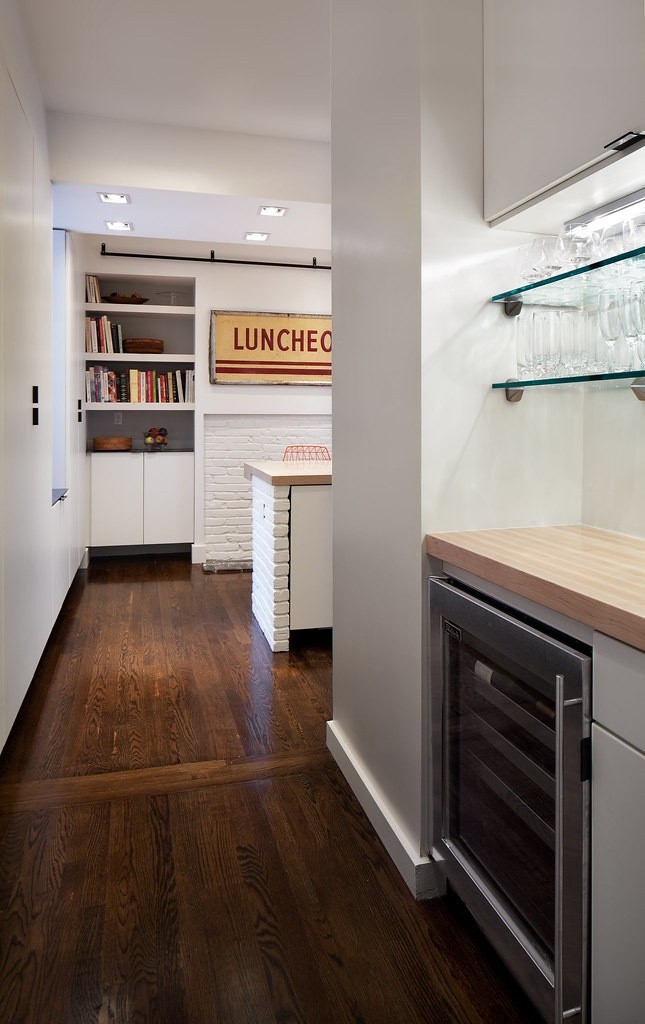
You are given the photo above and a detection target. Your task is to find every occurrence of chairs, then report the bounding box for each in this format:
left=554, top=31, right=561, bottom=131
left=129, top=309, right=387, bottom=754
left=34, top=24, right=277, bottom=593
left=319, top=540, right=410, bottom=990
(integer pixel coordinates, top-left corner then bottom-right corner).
left=283, top=446, right=331, bottom=462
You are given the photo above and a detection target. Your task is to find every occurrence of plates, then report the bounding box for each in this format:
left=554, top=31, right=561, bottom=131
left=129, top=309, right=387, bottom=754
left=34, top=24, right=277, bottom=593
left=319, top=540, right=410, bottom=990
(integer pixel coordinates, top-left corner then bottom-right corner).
left=102, top=296, right=149, bottom=304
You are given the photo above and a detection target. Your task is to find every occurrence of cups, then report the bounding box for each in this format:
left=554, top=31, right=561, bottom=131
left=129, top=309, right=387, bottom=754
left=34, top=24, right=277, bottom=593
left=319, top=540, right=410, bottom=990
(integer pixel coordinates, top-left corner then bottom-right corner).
left=517, top=309, right=598, bottom=381
left=520, top=217, right=645, bottom=285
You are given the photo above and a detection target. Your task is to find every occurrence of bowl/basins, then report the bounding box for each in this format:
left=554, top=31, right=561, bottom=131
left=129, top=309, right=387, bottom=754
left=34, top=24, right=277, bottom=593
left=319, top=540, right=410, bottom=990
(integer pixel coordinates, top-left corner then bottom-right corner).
left=142, top=432, right=168, bottom=450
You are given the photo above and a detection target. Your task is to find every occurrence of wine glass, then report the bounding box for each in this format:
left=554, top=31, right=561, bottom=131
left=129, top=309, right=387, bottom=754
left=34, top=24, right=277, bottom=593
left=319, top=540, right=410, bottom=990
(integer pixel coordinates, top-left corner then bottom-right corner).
left=599, top=288, right=645, bottom=372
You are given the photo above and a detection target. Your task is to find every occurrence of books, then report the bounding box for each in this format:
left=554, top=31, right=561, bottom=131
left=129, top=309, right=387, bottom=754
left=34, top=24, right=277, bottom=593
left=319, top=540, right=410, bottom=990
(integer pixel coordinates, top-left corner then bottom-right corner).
left=85, top=315, right=123, bottom=354
left=85, top=276, right=101, bottom=303
left=85, top=365, right=194, bottom=403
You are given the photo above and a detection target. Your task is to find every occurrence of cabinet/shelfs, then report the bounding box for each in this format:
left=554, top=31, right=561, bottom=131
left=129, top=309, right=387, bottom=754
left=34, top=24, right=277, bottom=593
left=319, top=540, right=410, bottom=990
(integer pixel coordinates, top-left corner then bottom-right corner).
left=590, top=633, right=645, bottom=1024
left=83, top=270, right=195, bottom=557
left=479, top=0, right=645, bottom=230
left=490, top=245, right=645, bottom=404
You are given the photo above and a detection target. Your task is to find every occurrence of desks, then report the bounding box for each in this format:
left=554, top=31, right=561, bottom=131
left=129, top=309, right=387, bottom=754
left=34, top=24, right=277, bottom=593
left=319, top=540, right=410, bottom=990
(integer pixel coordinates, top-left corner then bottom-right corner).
left=244, top=459, right=334, bottom=654
